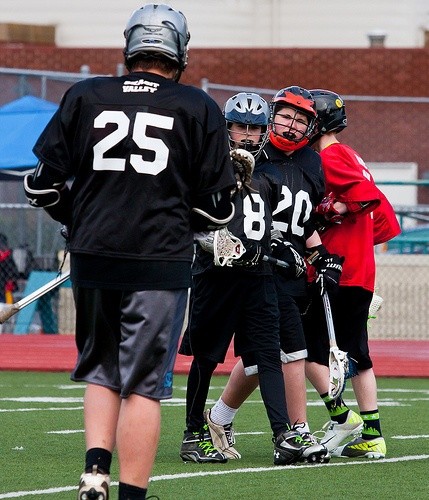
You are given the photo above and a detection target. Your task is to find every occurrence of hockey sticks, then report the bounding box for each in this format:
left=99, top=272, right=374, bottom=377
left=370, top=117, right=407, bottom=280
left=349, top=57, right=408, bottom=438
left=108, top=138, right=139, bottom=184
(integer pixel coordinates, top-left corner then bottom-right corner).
left=194, top=226, right=288, bottom=269
left=323, top=291, right=353, bottom=399
left=0, top=147, right=256, bottom=325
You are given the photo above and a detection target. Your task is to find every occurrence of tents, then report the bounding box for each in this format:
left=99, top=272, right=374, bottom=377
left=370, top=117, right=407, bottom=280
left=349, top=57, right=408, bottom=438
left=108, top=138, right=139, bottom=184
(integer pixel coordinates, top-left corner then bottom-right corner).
left=0, top=95, right=60, bottom=169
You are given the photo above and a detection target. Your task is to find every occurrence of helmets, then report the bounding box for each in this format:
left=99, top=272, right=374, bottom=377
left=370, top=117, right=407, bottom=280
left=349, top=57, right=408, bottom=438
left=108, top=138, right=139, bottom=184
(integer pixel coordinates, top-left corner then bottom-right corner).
left=308, top=89, right=348, bottom=145
left=222, top=93, right=270, bottom=162
left=269, top=86, right=318, bottom=153
left=123, top=4, right=190, bottom=69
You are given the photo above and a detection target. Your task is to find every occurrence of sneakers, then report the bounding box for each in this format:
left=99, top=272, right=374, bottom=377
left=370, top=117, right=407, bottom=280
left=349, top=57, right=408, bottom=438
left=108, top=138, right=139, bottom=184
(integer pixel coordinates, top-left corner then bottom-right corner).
left=272, top=418, right=328, bottom=467
left=204, top=408, right=242, bottom=460
left=292, top=430, right=332, bottom=465
left=322, top=409, right=363, bottom=454
left=179, top=425, right=228, bottom=463
left=329, top=435, right=387, bottom=459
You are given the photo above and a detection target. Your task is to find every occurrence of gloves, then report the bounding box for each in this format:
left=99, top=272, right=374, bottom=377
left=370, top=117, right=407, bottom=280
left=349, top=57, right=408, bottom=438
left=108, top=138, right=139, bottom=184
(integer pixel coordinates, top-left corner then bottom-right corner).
left=271, top=238, right=307, bottom=281
left=233, top=234, right=266, bottom=270
left=312, top=254, right=345, bottom=296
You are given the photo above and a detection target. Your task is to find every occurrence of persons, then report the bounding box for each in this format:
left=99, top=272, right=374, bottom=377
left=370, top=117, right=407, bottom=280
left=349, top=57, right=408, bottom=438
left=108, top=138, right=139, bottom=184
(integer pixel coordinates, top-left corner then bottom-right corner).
left=24, top=5, right=237, bottom=500
left=178, top=93, right=327, bottom=466
left=202, top=86, right=345, bottom=465
left=305, top=89, right=401, bottom=458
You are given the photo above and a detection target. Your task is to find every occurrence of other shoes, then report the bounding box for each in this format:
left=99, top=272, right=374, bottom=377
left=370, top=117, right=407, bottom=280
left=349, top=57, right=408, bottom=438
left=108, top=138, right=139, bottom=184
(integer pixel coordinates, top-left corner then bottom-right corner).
left=77, top=465, right=111, bottom=500
